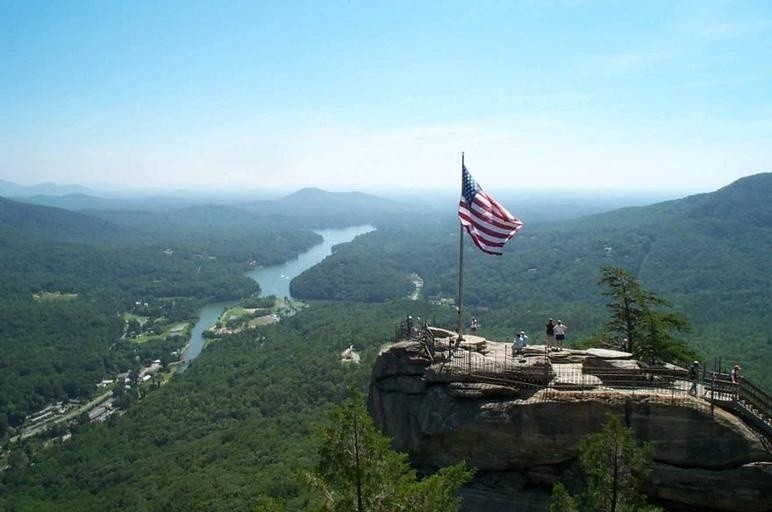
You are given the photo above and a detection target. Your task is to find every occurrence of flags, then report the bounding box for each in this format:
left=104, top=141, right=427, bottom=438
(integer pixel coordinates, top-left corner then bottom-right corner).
left=457, top=165, right=524, bottom=256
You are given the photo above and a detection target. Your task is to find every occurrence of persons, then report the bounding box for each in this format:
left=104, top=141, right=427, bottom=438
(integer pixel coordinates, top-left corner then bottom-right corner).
left=511, top=331, right=528, bottom=355
left=545, top=318, right=568, bottom=351
left=471, top=317, right=477, bottom=336
left=621, top=339, right=630, bottom=352
left=688, top=361, right=699, bottom=396
left=731, top=365, right=741, bottom=384
left=406, top=315, right=421, bottom=334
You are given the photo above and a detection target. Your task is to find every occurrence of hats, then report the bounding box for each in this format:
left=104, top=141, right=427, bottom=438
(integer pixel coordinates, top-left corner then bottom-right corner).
left=734, top=365, right=741, bottom=370
